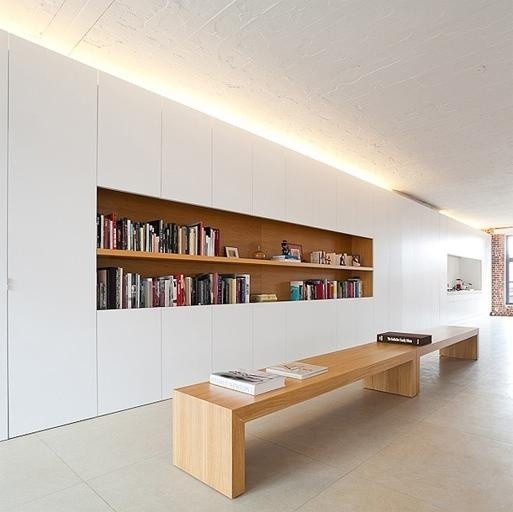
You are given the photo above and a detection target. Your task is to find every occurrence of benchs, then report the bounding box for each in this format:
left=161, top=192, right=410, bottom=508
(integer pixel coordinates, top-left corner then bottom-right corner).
left=173, top=326, right=479, bottom=500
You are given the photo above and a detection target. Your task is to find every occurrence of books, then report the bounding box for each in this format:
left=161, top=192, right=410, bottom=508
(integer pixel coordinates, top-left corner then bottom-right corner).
left=310, top=251, right=354, bottom=266
left=266, top=361, right=328, bottom=381
left=289, top=276, right=363, bottom=301
left=97, top=267, right=250, bottom=310
left=209, top=366, right=287, bottom=395
left=97, top=212, right=220, bottom=257
left=377, top=332, right=432, bottom=346
left=250, top=294, right=278, bottom=302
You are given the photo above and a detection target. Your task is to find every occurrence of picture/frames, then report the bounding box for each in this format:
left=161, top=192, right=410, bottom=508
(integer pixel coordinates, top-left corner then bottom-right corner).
left=287, top=243, right=303, bottom=261
left=224, top=246, right=239, bottom=258
